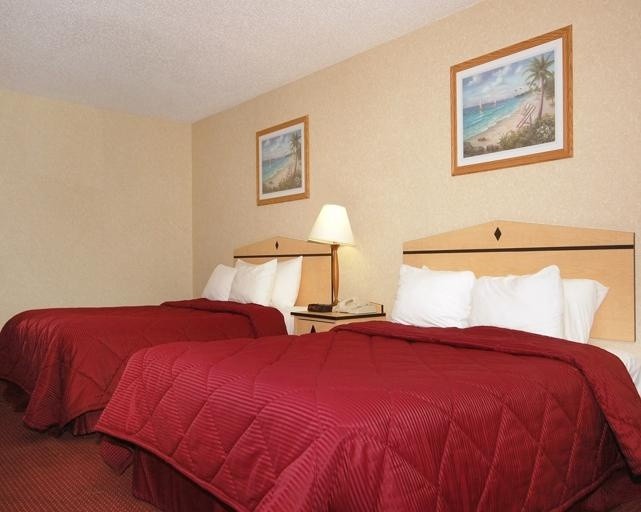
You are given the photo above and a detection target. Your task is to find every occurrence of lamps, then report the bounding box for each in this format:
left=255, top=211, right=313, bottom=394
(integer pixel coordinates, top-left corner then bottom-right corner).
left=306, top=204, right=354, bottom=306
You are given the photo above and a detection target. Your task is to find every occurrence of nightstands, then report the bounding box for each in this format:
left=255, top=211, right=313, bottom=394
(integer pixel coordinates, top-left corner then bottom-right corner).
left=291, top=310, right=386, bottom=336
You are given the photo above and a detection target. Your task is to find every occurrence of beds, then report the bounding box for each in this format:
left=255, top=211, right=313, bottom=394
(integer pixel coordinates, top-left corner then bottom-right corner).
left=95, top=219, right=641, bottom=511
left=0, top=235, right=332, bottom=436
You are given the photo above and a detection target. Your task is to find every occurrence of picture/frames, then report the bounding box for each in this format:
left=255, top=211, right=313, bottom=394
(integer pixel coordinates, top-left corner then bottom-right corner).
left=255, top=114, right=310, bottom=206
left=449, top=23, right=574, bottom=179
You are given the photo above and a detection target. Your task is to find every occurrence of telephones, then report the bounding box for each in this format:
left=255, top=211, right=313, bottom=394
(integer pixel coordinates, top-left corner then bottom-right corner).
left=331, top=297, right=377, bottom=314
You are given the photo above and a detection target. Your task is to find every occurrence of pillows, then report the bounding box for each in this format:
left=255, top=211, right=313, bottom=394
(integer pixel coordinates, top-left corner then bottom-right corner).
left=198, top=255, right=304, bottom=312
left=387, top=265, right=610, bottom=345
